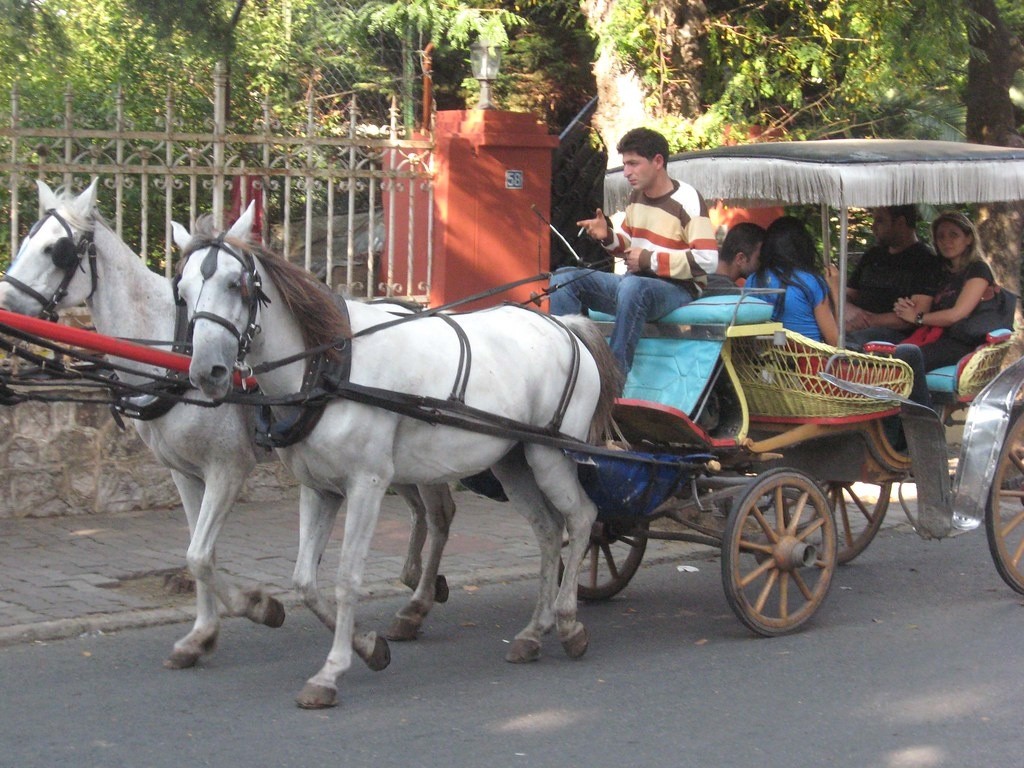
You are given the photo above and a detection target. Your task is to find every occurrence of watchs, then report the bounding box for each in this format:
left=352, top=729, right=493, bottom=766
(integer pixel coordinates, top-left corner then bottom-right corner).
left=915, top=312, right=923, bottom=325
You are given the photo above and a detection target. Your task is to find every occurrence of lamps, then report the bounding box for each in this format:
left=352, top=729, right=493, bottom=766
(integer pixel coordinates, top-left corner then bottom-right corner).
left=470, top=37, right=503, bottom=109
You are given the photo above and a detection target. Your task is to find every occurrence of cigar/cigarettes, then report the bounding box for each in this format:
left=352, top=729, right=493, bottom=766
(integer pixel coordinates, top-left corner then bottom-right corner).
left=577, top=225, right=586, bottom=237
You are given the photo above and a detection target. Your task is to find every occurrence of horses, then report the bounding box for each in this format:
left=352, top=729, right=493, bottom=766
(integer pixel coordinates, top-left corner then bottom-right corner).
left=170, top=200, right=626, bottom=710
left=1, top=176, right=459, bottom=670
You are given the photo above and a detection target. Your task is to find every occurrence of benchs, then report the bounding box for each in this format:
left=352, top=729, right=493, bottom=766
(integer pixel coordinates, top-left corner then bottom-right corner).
left=924, top=327, right=1017, bottom=405
left=720, top=327, right=912, bottom=425
left=588, top=286, right=783, bottom=341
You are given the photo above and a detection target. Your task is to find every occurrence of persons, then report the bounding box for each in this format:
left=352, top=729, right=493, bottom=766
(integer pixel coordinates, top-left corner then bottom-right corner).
left=701, top=221, right=766, bottom=298
left=742, top=215, right=933, bottom=448
left=866, top=209, right=999, bottom=375
left=548, top=128, right=719, bottom=400
left=828, top=200, right=940, bottom=360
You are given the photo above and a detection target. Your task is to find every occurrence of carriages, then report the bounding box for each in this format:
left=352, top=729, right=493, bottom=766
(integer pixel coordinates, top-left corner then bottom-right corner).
left=0, top=138, right=1023, bottom=704
